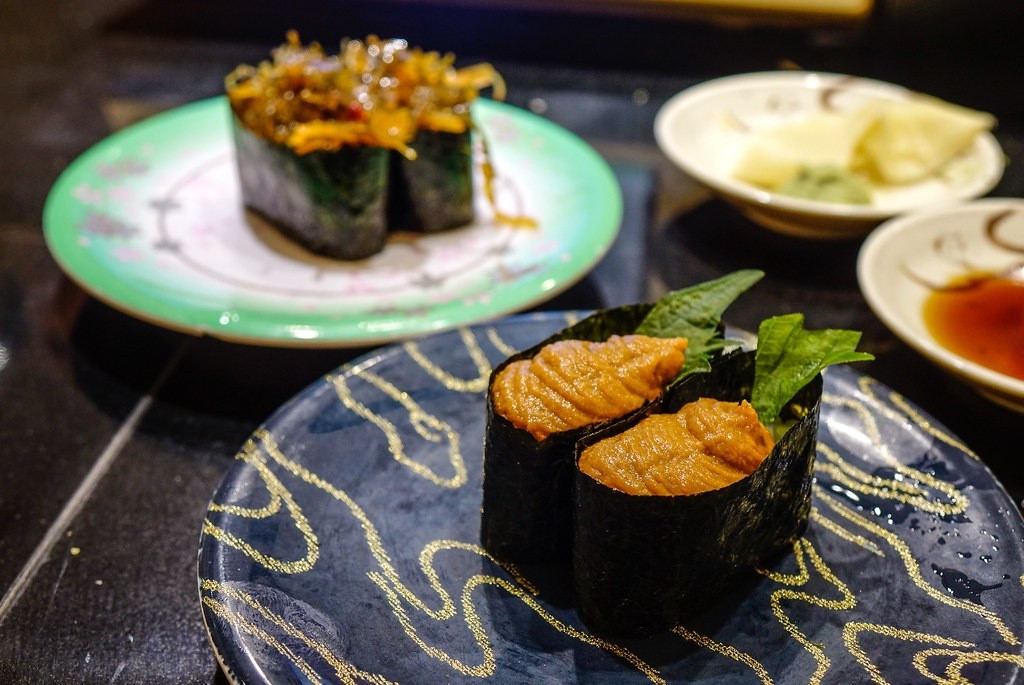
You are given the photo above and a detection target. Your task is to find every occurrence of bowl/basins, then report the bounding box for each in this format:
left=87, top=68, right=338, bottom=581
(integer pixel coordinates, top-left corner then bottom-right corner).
left=654, top=71, right=1006, bottom=239
left=856, top=198, right=1024, bottom=412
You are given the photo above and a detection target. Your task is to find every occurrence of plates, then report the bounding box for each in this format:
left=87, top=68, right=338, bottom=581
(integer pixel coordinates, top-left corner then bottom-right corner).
left=200, top=310, right=1024, bottom=685
left=43, top=95, right=624, bottom=349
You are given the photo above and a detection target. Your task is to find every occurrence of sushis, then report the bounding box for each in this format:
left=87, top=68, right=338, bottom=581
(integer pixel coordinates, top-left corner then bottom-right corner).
left=480, top=269, right=875, bottom=671
left=225, top=29, right=534, bottom=261
